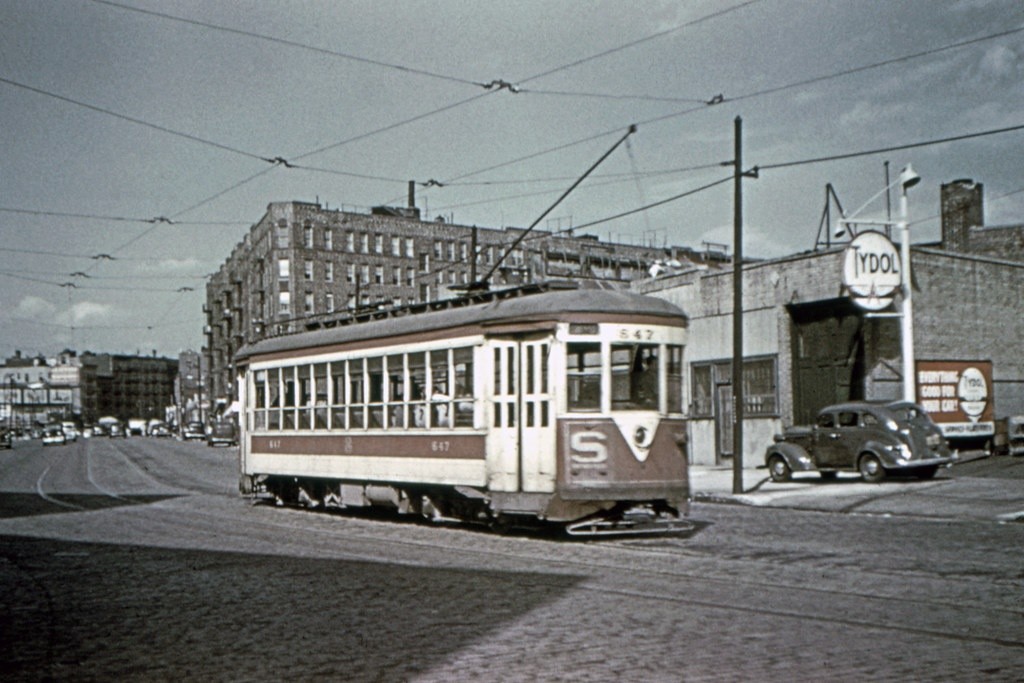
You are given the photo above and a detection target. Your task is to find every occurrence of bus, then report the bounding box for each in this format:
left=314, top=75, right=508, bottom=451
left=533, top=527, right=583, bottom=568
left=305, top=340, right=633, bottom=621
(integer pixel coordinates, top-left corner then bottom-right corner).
left=232, top=123, right=698, bottom=536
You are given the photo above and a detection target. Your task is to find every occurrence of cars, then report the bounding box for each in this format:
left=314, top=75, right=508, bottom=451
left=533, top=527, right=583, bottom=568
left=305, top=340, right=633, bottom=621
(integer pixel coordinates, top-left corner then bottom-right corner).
left=0, top=425, right=14, bottom=449
left=40, top=415, right=173, bottom=445
left=760, top=398, right=962, bottom=484
left=180, top=420, right=206, bottom=441
left=203, top=422, right=236, bottom=447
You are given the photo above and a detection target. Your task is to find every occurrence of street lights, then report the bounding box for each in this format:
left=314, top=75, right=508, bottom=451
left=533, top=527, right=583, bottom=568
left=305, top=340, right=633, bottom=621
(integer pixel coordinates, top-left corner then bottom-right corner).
left=832, top=161, right=924, bottom=419
